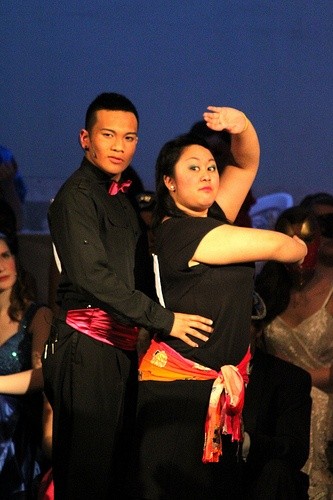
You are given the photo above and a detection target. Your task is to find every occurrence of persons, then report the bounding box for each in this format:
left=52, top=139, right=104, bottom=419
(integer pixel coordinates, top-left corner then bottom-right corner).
left=47, top=92, right=215, bottom=500
left=251, top=205, right=333, bottom=500
left=0, top=157, right=26, bottom=260
left=188, top=120, right=257, bottom=228
left=0, top=234, right=53, bottom=500
left=300, top=192, right=333, bottom=268
left=131, top=106, right=308, bottom=500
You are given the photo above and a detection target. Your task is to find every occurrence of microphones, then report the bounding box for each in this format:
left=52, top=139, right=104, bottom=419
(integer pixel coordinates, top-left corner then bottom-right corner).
left=85, top=148, right=96, bottom=158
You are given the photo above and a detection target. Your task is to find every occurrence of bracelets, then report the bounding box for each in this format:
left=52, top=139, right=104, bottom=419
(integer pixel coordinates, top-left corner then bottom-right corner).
left=240, top=111, right=248, bottom=132
